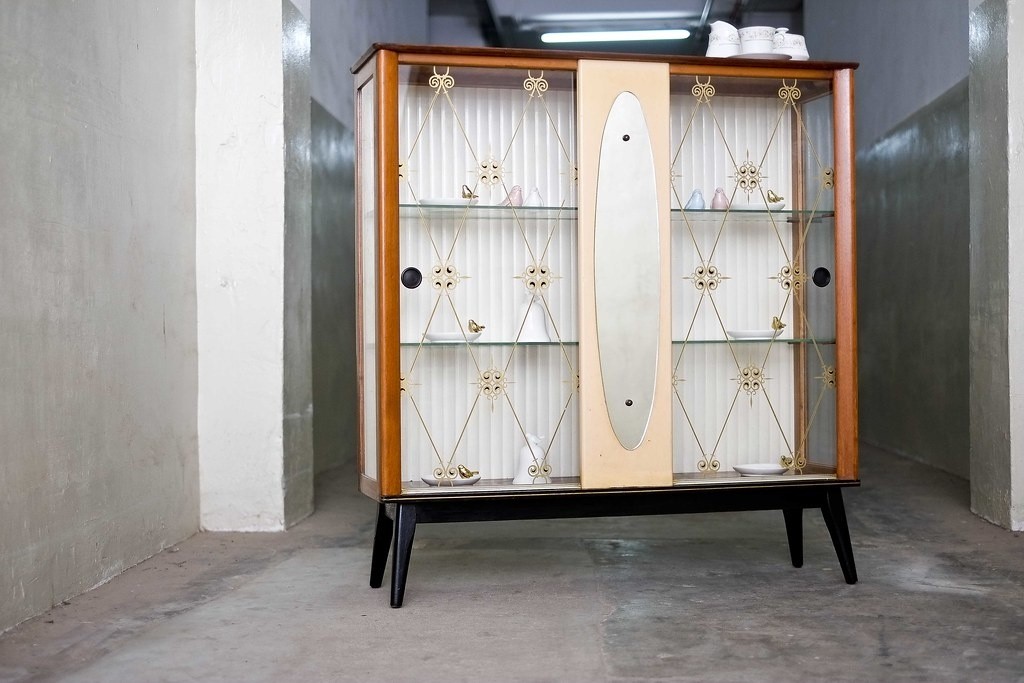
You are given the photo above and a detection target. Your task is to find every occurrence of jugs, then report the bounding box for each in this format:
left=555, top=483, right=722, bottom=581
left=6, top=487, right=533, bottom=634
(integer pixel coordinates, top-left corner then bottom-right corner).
left=705, top=20, right=741, bottom=58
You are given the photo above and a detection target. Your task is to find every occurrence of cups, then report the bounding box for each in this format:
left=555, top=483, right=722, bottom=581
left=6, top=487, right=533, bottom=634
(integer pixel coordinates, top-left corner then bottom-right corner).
left=737, top=26, right=785, bottom=53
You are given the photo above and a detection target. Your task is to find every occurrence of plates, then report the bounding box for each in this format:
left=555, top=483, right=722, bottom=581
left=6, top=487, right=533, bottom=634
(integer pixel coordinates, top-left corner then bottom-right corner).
left=732, top=464, right=789, bottom=477
left=726, top=330, right=784, bottom=340
left=726, top=53, right=792, bottom=59
left=748, top=203, right=786, bottom=210
left=418, top=198, right=479, bottom=205
left=421, top=331, right=482, bottom=343
left=420, top=474, right=481, bottom=486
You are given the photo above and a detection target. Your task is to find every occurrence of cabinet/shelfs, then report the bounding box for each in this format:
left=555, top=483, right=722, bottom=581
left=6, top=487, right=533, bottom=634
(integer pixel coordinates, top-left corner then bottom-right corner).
left=350, top=42, right=860, bottom=607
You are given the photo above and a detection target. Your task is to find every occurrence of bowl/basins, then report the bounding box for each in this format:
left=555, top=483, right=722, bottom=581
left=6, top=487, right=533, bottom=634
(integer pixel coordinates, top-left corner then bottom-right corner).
left=773, top=27, right=810, bottom=61
